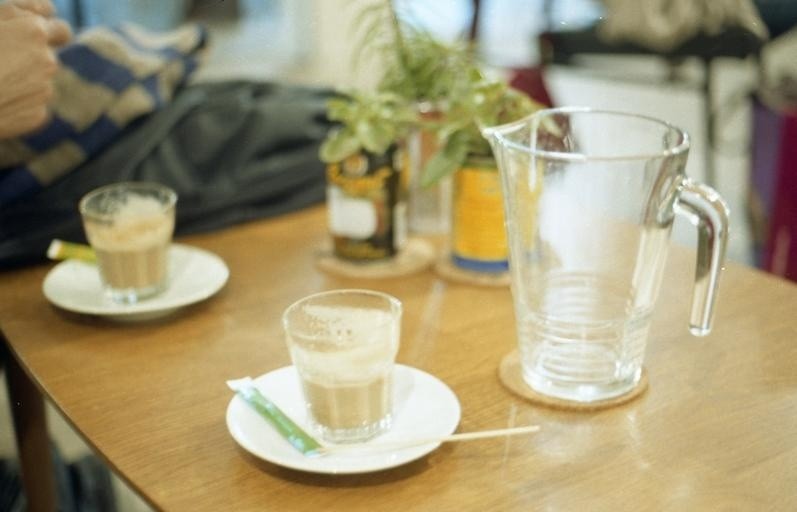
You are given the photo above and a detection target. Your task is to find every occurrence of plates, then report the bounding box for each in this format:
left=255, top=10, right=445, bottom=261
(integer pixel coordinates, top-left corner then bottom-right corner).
left=227, top=363, right=461, bottom=474
left=42, top=242, right=229, bottom=322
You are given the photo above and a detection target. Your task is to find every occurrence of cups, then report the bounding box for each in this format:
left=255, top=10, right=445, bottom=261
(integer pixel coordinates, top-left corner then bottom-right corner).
left=484, top=107, right=728, bottom=401
left=283, top=290, right=401, bottom=442
left=79, top=182, right=178, bottom=303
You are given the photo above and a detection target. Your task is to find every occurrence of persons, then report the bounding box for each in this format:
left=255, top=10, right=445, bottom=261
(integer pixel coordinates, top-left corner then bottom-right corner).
left=0, top=1, right=70, bottom=141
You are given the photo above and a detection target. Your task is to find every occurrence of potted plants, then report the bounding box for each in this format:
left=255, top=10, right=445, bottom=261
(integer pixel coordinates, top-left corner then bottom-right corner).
left=319, top=93, right=415, bottom=262
left=347, top=1, right=487, bottom=200
left=422, top=81, right=542, bottom=274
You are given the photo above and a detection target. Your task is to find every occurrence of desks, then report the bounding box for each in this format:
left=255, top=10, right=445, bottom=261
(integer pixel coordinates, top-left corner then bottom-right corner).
left=0, top=200, right=797, bottom=512
